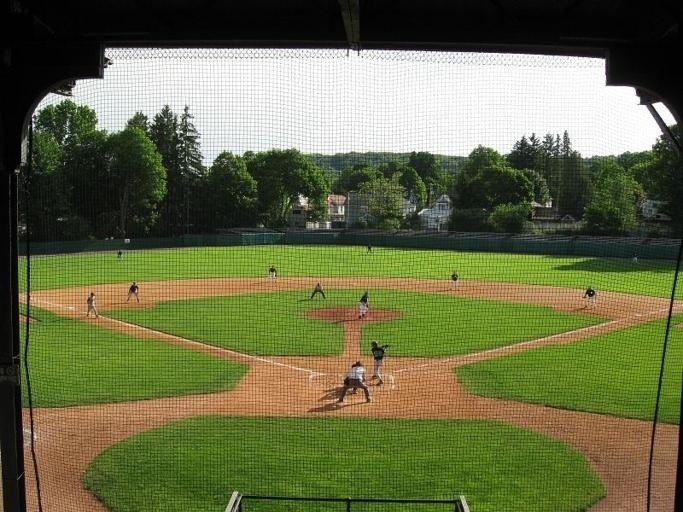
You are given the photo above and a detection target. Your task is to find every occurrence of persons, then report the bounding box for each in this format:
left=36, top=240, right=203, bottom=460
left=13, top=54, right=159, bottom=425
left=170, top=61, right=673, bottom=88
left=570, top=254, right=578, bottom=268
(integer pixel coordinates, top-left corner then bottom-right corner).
left=371, top=340, right=384, bottom=386
left=451, top=271, right=458, bottom=286
left=311, top=283, right=326, bottom=299
left=269, top=265, right=277, bottom=277
left=358, top=291, right=369, bottom=319
left=339, top=360, right=372, bottom=403
left=584, top=285, right=595, bottom=307
left=87, top=292, right=99, bottom=318
left=367, top=242, right=372, bottom=254
left=632, top=251, right=639, bottom=263
left=343, top=364, right=358, bottom=394
left=126, top=281, right=139, bottom=303
